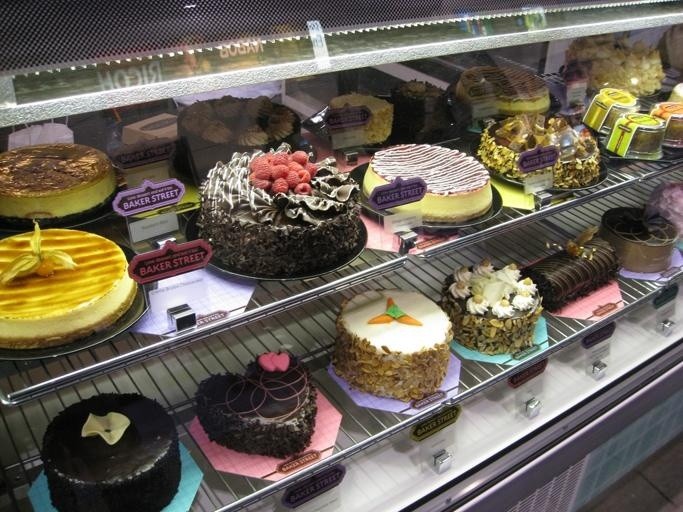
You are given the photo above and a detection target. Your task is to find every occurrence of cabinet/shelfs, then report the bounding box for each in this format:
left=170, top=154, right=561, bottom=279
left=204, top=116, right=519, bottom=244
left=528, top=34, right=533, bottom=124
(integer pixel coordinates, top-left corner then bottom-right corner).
left=1, top=0, right=682, bottom=512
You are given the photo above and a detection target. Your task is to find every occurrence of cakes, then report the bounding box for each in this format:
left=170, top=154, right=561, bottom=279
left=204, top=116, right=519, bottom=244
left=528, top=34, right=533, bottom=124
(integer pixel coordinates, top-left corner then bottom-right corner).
left=0, top=35, right=682, bottom=363
left=40, top=181, right=682, bottom=512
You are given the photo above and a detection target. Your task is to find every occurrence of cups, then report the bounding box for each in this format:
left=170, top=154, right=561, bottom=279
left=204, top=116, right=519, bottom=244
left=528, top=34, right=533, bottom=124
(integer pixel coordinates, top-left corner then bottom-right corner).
left=522, top=6, right=546, bottom=30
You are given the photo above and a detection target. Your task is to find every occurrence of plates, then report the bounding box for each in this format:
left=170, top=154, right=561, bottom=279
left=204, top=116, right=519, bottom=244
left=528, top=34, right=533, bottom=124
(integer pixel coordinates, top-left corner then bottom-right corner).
left=0, top=245, right=145, bottom=361
left=1, top=165, right=128, bottom=234
left=468, top=138, right=609, bottom=190
left=344, top=160, right=503, bottom=231
left=557, top=64, right=659, bottom=99
left=444, top=87, right=563, bottom=134
left=167, top=141, right=317, bottom=179
left=188, top=209, right=369, bottom=276
